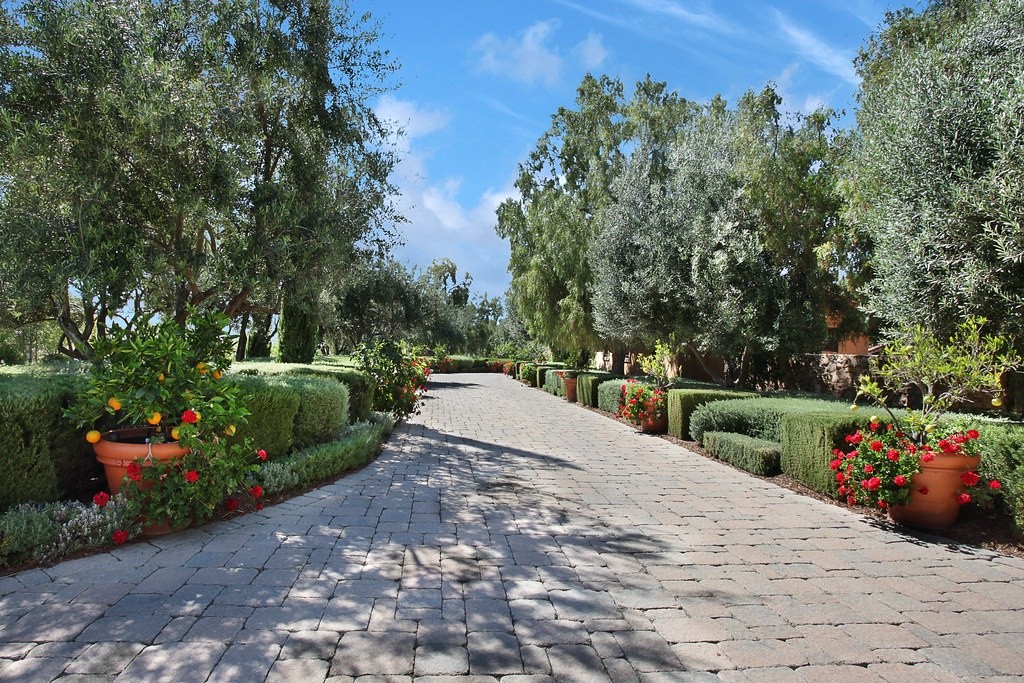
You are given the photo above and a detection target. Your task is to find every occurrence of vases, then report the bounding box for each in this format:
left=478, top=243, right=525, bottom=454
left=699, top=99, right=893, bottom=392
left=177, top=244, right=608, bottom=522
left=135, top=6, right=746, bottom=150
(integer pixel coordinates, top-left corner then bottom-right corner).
left=562, top=378, right=579, bottom=403
left=880, top=448, right=982, bottom=527
left=91, top=424, right=212, bottom=536
left=640, top=410, right=669, bottom=435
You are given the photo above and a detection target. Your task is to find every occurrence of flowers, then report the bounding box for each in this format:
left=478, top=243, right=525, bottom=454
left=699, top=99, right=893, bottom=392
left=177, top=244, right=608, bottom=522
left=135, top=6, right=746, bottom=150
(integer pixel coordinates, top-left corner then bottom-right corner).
left=61, top=320, right=266, bottom=544
left=557, top=348, right=586, bottom=379
left=614, top=338, right=674, bottom=425
left=824, top=316, right=1022, bottom=511
